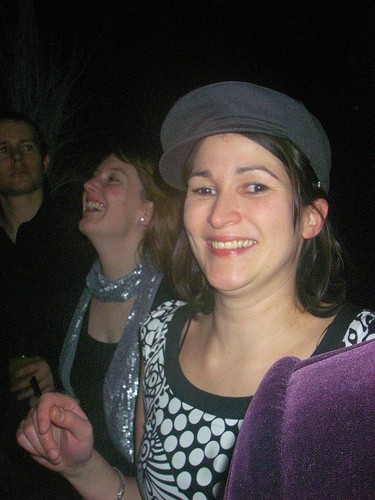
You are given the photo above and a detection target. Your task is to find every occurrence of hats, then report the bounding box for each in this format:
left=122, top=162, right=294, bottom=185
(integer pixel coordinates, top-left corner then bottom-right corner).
left=158, top=81, right=331, bottom=195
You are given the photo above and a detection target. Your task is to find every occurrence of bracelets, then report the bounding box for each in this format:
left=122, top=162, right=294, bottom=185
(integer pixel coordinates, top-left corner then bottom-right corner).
left=111, top=467, right=126, bottom=500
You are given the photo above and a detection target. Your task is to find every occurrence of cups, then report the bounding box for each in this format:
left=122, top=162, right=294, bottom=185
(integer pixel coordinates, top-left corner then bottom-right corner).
left=7, top=337, right=35, bottom=425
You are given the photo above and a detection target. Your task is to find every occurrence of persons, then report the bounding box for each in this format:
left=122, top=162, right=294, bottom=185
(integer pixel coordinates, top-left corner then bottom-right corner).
left=224, top=335, right=375, bottom=498
left=57, top=136, right=185, bottom=477
left=0, top=110, right=100, bottom=500
left=16, top=81, right=374, bottom=500
